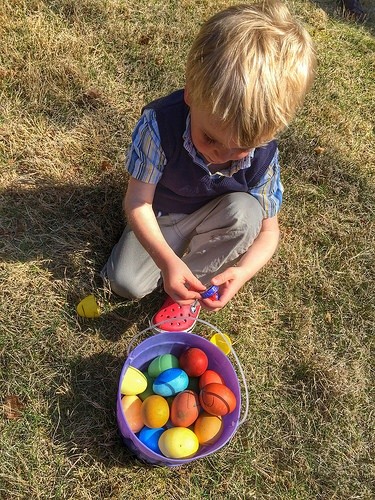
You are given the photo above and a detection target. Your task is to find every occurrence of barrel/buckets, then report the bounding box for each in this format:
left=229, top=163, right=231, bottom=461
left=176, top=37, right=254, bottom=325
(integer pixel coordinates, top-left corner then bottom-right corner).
left=115, top=317, right=249, bottom=467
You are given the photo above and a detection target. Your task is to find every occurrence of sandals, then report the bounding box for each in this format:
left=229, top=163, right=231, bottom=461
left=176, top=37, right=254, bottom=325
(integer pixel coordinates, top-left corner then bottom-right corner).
left=150, top=297, right=201, bottom=333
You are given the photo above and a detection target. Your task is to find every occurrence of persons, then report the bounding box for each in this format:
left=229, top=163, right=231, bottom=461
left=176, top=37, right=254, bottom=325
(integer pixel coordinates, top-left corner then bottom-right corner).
left=106, top=1, right=315, bottom=338
